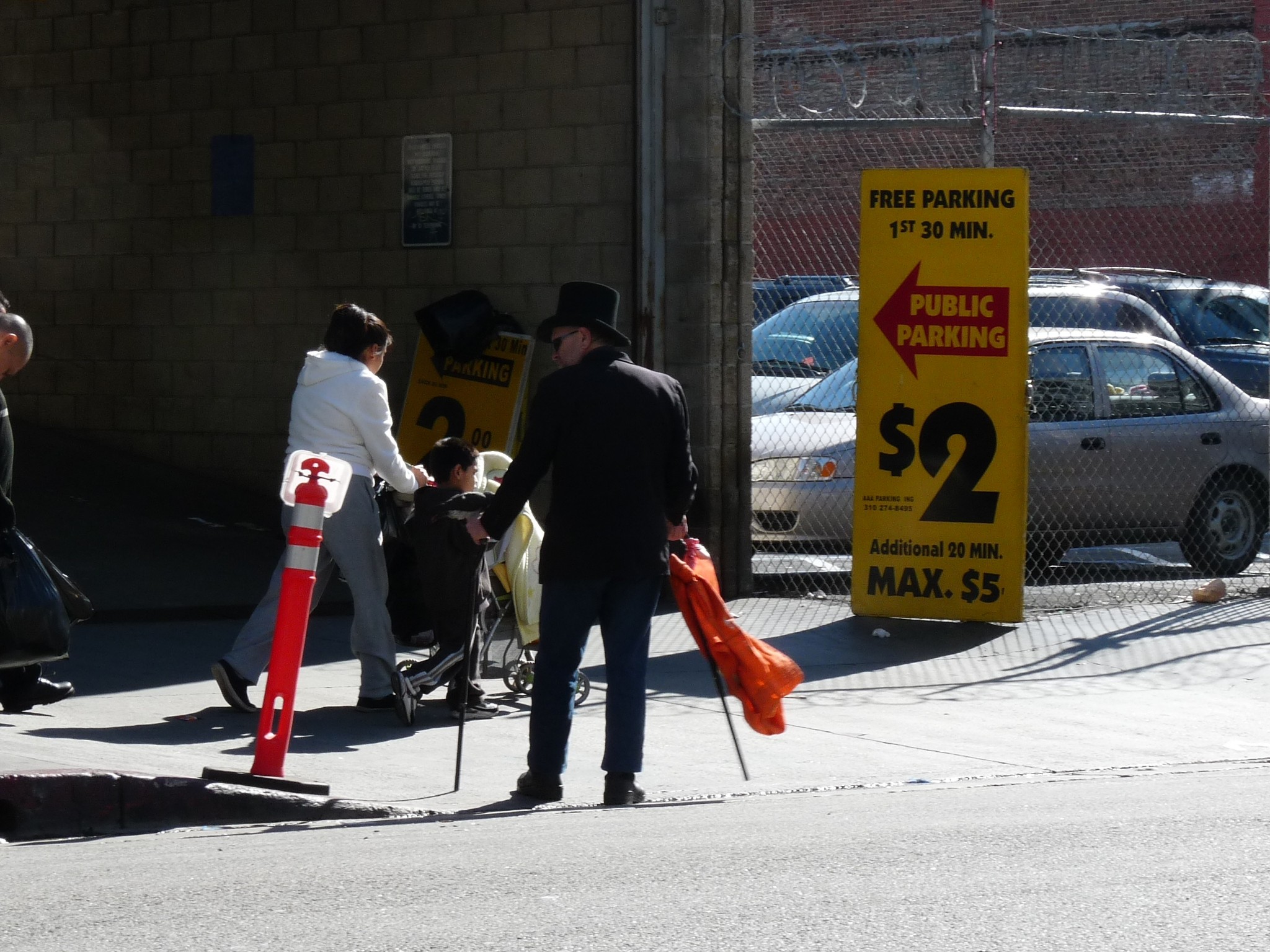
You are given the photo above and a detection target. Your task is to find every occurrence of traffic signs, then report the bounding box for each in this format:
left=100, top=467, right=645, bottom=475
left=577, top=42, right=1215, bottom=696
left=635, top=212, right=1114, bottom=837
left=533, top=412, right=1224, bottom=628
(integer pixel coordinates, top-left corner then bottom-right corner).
left=850, top=168, right=1027, bottom=625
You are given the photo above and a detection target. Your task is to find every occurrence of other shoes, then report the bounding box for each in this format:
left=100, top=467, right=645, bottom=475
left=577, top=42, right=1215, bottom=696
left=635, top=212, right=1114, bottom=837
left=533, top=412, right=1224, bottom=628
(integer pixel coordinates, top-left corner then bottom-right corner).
left=602, top=772, right=652, bottom=807
left=354, top=694, right=400, bottom=712
left=209, top=658, right=259, bottom=714
left=517, top=770, right=571, bottom=803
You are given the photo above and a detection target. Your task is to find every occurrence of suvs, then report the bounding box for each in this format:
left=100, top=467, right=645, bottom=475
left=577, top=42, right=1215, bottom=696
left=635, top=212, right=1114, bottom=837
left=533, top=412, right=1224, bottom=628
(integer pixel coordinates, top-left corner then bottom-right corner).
left=751, top=267, right=1270, bottom=415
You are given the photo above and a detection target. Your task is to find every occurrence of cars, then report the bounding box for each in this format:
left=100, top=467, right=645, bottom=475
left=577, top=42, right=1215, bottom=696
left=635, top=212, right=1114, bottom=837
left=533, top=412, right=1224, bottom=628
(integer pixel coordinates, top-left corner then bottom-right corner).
left=743, top=328, right=1270, bottom=575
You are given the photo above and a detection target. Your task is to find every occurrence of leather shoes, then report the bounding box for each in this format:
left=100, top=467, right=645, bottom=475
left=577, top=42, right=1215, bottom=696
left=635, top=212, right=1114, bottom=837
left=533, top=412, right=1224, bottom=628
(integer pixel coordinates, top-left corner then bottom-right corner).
left=0, top=677, right=74, bottom=713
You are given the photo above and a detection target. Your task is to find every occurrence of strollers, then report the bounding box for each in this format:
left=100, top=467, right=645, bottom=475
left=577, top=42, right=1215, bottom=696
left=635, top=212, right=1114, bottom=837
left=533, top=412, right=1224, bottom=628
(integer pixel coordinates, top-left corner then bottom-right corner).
left=376, top=453, right=590, bottom=708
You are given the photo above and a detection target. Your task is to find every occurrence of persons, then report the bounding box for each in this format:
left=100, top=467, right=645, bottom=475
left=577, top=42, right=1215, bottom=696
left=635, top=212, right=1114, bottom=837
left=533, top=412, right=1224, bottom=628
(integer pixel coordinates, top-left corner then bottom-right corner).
left=391, top=434, right=505, bottom=726
left=210, top=304, right=428, bottom=713
left=0, top=290, right=76, bottom=713
left=463, top=281, right=700, bottom=808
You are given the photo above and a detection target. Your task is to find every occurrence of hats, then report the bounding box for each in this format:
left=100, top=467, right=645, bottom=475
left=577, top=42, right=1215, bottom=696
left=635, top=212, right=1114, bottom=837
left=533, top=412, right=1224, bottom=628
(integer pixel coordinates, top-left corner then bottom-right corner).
left=535, top=281, right=634, bottom=348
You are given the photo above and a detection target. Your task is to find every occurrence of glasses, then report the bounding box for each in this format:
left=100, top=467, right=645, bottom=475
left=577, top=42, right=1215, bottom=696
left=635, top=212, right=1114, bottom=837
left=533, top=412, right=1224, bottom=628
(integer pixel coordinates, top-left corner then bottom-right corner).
left=550, top=331, right=578, bottom=350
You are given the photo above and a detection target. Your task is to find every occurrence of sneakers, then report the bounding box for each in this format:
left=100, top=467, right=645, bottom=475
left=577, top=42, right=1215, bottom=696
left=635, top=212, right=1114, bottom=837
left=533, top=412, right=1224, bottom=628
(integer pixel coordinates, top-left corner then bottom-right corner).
left=390, top=670, right=422, bottom=728
left=449, top=697, right=499, bottom=723
